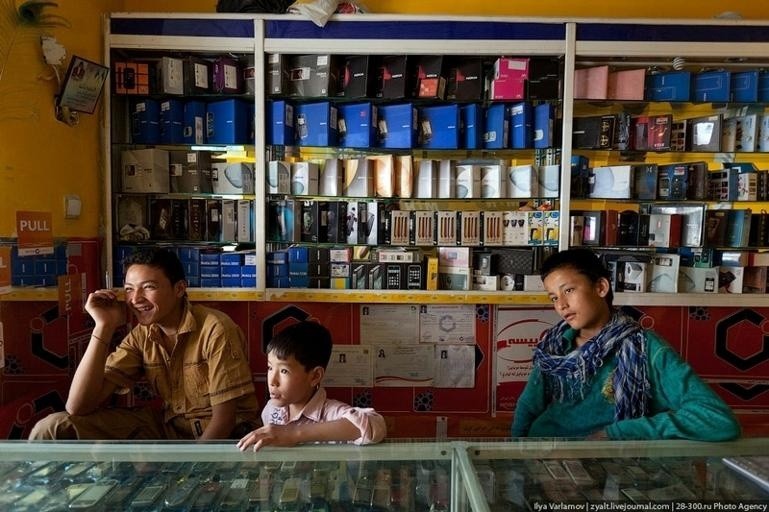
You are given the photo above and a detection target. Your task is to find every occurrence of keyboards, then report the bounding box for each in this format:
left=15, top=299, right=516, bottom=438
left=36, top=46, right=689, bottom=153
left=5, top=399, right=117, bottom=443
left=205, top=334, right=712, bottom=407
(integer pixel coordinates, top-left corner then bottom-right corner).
left=721, top=455, right=768, bottom=489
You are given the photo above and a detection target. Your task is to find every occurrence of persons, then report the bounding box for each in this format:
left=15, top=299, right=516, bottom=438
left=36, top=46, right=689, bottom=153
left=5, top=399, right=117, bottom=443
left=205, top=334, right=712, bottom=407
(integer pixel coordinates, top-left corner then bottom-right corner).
left=511, top=246, right=742, bottom=443
left=27, top=247, right=262, bottom=442
left=237, top=321, right=388, bottom=454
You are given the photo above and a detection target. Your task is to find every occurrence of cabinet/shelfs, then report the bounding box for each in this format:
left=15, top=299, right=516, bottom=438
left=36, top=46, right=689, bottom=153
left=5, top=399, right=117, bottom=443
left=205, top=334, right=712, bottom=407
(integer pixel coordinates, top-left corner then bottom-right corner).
left=0, top=438, right=769, bottom=512
left=103, top=12, right=769, bottom=290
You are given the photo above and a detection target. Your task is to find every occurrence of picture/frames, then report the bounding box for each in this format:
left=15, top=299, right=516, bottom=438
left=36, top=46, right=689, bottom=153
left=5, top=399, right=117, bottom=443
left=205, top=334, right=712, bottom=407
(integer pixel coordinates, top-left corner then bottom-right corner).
left=56, top=55, right=110, bottom=114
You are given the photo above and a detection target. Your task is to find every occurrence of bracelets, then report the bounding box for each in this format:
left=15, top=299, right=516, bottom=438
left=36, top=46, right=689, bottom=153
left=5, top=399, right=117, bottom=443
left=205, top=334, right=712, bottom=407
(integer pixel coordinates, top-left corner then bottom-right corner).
left=90, top=332, right=112, bottom=350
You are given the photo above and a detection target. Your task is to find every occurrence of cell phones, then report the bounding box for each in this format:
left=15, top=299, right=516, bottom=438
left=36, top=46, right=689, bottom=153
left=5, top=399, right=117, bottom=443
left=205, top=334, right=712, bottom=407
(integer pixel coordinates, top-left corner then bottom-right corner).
left=472, top=451, right=689, bottom=511
left=407, top=265, right=422, bottom=288
left=0, top=458, right=451, bottom=512
left=386, top=265, right=402, bottom=289
